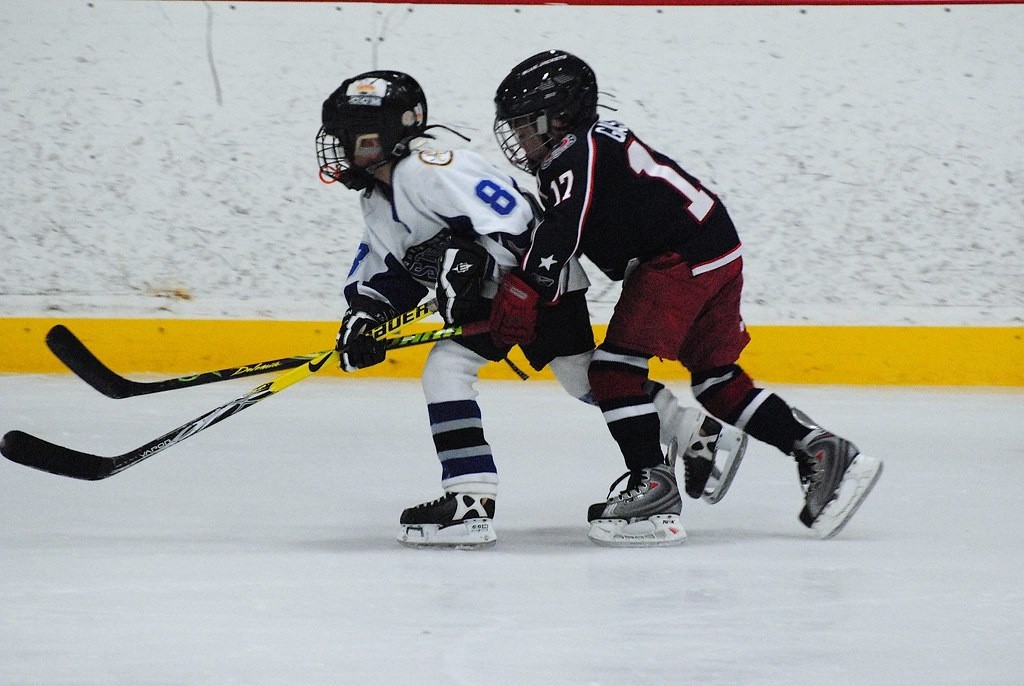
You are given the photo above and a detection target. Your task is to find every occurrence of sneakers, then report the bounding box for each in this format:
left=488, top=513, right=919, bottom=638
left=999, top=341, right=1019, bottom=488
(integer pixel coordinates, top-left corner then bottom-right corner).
left=786, top=406, right=882, bottom=539
left=398, top=490, right=498, bottom=551
left=681, top=416, right=747, bottom=504
left=587, top=437, right=688, bottom=548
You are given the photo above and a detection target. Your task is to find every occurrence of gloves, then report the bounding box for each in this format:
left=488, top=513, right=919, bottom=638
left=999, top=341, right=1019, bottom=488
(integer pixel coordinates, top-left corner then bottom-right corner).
left=488, top=271, right=538, bottom=348
left=434, top=242, right=493, bottom=326
left=335, top=293, right=399, bottom=373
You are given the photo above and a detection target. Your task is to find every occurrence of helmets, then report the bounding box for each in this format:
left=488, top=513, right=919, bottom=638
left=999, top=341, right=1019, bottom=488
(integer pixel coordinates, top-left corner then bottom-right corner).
left=315, top=71, right=430, bottom=191
left=493, top=49, right=597, bottom=175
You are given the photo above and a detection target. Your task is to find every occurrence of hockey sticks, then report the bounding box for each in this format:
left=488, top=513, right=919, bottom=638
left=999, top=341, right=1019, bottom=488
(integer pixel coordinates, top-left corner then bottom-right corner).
left=0, top=298, right=440, bottom=483
left=44, top=322, right=490, bottom=401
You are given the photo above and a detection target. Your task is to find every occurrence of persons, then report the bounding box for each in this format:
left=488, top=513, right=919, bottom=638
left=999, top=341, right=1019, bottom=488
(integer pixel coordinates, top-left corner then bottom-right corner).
left=490, top=50, right=882, bottom=549
left=314, top=69, right=751, bottom=551
